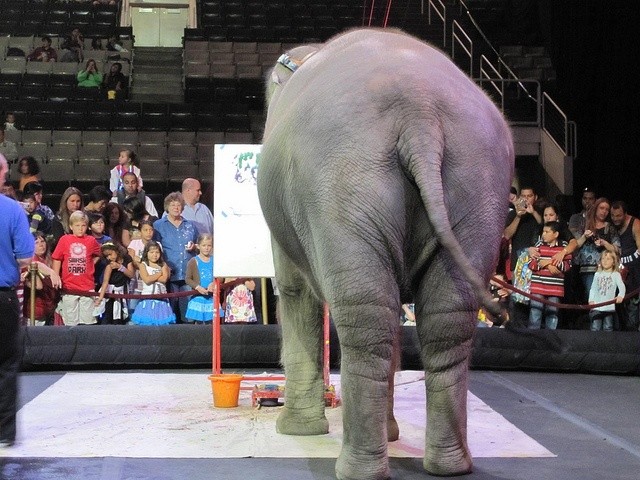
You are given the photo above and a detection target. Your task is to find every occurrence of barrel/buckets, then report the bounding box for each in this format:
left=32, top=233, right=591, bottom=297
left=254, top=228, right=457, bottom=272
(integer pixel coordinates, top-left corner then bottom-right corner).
left=207, top=373, right=244, bottom=407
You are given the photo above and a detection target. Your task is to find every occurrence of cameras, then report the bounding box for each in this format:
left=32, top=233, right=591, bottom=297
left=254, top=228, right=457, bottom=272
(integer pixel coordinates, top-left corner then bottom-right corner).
left=518, top=197, right=527, bottom=209
left=21, top=202, right=31, bottom=209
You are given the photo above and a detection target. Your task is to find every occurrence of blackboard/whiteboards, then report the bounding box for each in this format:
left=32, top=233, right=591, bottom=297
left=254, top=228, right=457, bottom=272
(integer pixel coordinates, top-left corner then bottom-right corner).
left=212, top=144, right=328, bottom=391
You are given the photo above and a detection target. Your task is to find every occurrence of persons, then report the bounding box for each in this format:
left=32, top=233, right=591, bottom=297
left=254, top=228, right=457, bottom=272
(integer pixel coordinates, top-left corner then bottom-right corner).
left=568, top=191, right=595, bottom=240
left=0, top=125, right=18, bottom=162
left=95, top=241, right=133, bottom=325
left=504, top=186, right=544, bottom=285
left=162, top=178, right=214, bottom=235
left=51, top=211, right=102, bottom=326
left=610, top=201, right=640, bottom=331
left=102, top=202, right=123, bottom=242
left=152, top=191, right=199, bottom=324
left=4, top=113, right=17, bottom=130
left=88, top=214, right=112, bottom=245
left=22, top=181, right=64, bottom=256
left=110, top=149, right=143, bottom=196
left=127, top=221, right=163, bottom=292
left=84, top=186, right=113, bottom=212
left=17, top=156, right=40, bottom=191
left=131, top=241, right=176, bottom=325
left=26, top=28, right=125, bottom=90
left=1, top=182, right=17, bottom=198
left=577, top=197, right=621, bottom=320
left=525, top=206, right=577, bottom=329
left=24, top=231, right=62, bottom=320
left=121, top=197, right=159, bottom=248
left=109, top=172, right=159, bottom=218
left=55, top=187, right=84, bottom=234
left=0, top=154, right=35, bottom=447
left=185, top=233, right=225, bottom=324
left=588, top=250, right=626, bottom=331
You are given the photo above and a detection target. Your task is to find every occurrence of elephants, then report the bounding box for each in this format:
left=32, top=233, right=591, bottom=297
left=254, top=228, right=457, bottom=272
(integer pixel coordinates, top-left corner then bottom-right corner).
left=256, top=27, right=515, bottom=480
